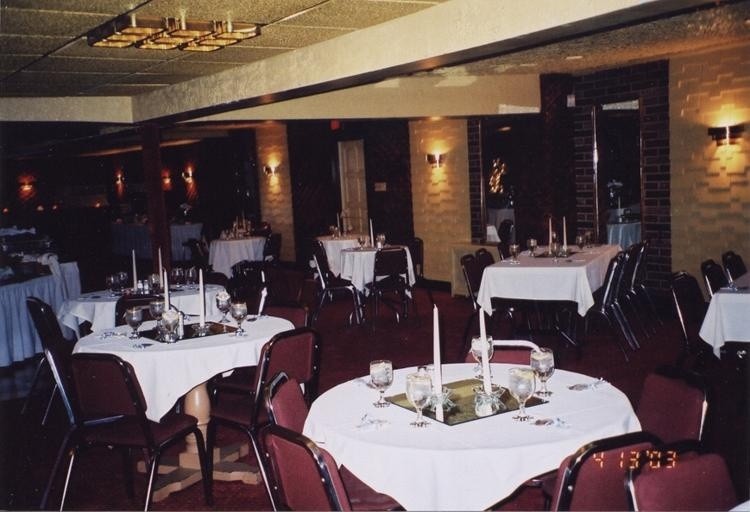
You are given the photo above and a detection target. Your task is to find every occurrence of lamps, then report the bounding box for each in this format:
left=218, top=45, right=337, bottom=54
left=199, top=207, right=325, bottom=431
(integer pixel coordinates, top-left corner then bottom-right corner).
left=708, top=122, right=745, bottom=147
left=260, top=164, right=281, bottom=177
left=425, top=152, right=449, bottom=169
left=85, top=11, right=262, bottom=53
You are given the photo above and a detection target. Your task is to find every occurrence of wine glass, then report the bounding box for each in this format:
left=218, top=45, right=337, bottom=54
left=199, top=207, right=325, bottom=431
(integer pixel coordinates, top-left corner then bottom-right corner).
left=107, top=265, right=248, bottom=341
left=507, top=228, right=597, bottom=266
left=327, top=224, right=387, bottom=251
left=370, top=335, right=558, bottom=426
